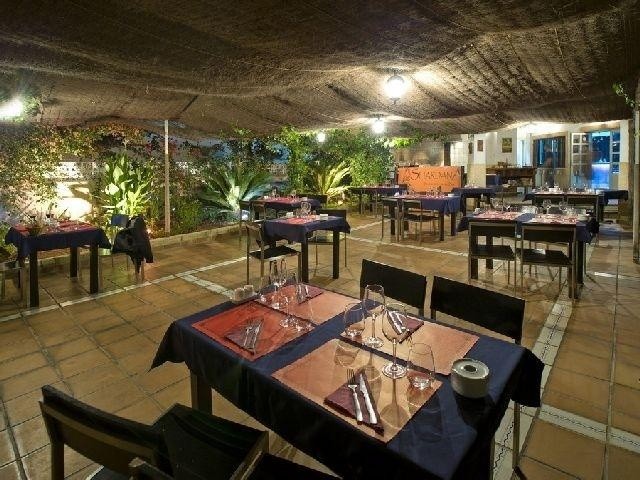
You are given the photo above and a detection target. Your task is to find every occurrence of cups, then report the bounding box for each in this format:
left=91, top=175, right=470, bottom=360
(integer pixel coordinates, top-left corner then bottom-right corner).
left=406, top=340, right=434, bottom=391
left=495, top=164, right=522, bottom=168
left=332, top=337, right=362, bottom=368
left=343, top=303, right=366, bottom=336
left=407, top=388, right=443, bottom=443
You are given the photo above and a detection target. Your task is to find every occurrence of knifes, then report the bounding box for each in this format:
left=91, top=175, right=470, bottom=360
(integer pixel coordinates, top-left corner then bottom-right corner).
left=360, top=372, right=380, bottom=426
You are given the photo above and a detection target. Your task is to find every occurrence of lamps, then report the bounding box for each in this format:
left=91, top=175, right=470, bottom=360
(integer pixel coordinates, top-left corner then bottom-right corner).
left=371, top=113, right=386, bottom=134
left=382, top=70, right=406, bottom=104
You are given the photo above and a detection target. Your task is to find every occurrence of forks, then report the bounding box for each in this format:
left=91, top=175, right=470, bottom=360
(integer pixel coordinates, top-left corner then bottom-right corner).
left=346, top=367, right=363, bottom=424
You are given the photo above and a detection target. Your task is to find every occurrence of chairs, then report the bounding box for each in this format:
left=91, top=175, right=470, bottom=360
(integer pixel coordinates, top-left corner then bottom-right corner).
left=35, top=381, right=268, bottom=480
left=76, top=211, right=150, bottom=287
left=430, top=273, right=526, bottom=480
left=345, top=162, right=630, bottom=301
left=242, top=449, right=343, bottom=480
left=355, top=255, right=427, bottom=320
left=231, top=191, right=351, bottom=287
left=0, top=249, right=29, bottom=313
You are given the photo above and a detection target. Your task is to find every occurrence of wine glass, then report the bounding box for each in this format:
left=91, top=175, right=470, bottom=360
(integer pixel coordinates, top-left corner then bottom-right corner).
left=379, top=380, right=415, bottom=448
left=276, top=202, right=329, bottom=223
left=379, top=302, right=409, bottom=382
left=231, top=257, right=317, bottom=353
left=368, top=178, right=392, bottom=188
left=474, top=197, right=589, bottom=227
left=466, top=178, right=476, bottom=189
left=533, top=180, right=603, bottom=197
left=8, top=200, right=83, bottom=235
left=264, top=188, right=308, bottom=204
left=360, top=284, right=385, bottom=348
left=400, top=184, right=455, bottom=199
left=354, top=349, right=382, bottom=412
